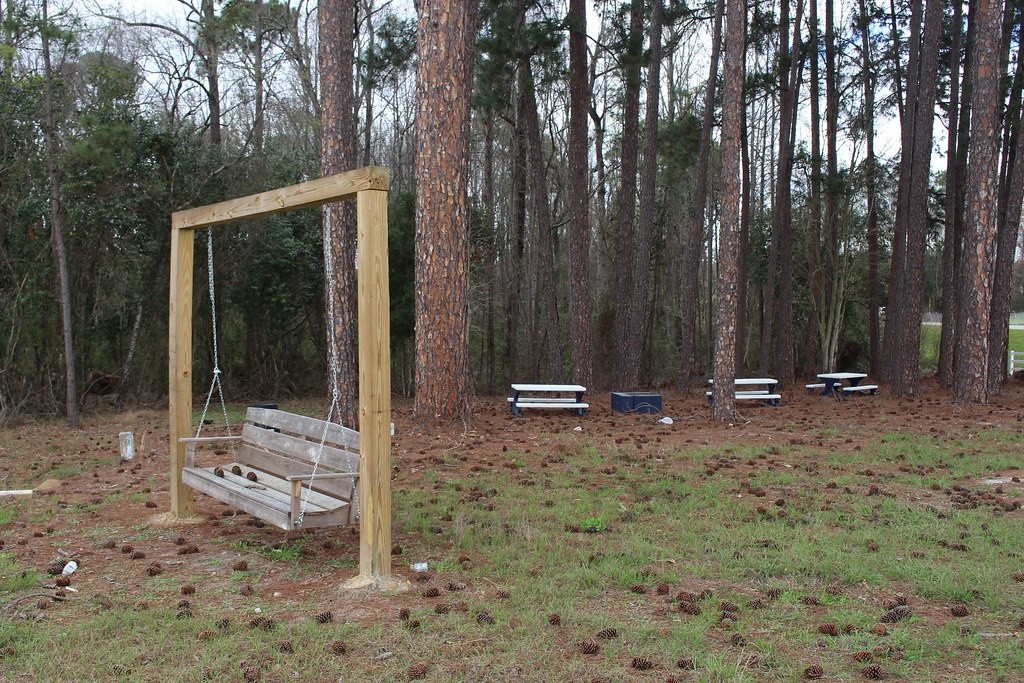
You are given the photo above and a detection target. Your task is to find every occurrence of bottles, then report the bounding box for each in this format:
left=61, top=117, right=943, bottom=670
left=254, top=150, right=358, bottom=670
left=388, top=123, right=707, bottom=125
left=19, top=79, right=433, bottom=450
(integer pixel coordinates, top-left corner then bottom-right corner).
left=62, top=561, right=78, bottom=576
left=410, top=562, right=428, bottom=573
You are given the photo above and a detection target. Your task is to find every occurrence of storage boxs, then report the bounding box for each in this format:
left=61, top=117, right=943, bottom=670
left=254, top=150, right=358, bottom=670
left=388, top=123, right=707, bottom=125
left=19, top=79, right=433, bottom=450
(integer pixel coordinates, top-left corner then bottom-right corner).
left=611, top=392, right=634, bottom=413
left=626, top=392, right=663, bottom=413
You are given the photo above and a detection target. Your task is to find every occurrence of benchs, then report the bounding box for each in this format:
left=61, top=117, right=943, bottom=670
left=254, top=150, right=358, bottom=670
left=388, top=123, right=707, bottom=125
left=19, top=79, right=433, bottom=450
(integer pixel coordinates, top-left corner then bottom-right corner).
left=516, top=403, right=590, bottom=409
left=735, top=394, right=782, bottom=399
left=507, top=398, right=577, bottom=402
left=706, top=390, right=769, bottom=396
left=842, top=384, right=878, bottom=392
left=804, top=383, right=841, bottom=388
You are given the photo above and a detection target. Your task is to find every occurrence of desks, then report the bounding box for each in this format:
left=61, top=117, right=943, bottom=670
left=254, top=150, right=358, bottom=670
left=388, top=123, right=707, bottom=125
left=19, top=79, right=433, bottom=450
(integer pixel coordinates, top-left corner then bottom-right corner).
left=510, top=383, right=587, bottom=416
left=708, top=377, right=778, bottom=406
left=816, top=373, right=868, bottom=397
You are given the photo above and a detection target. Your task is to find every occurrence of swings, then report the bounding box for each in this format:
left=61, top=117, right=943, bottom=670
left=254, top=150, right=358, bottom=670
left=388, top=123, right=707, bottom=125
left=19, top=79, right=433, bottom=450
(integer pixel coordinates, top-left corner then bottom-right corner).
left=181, top=204, right=365, bottom=535
